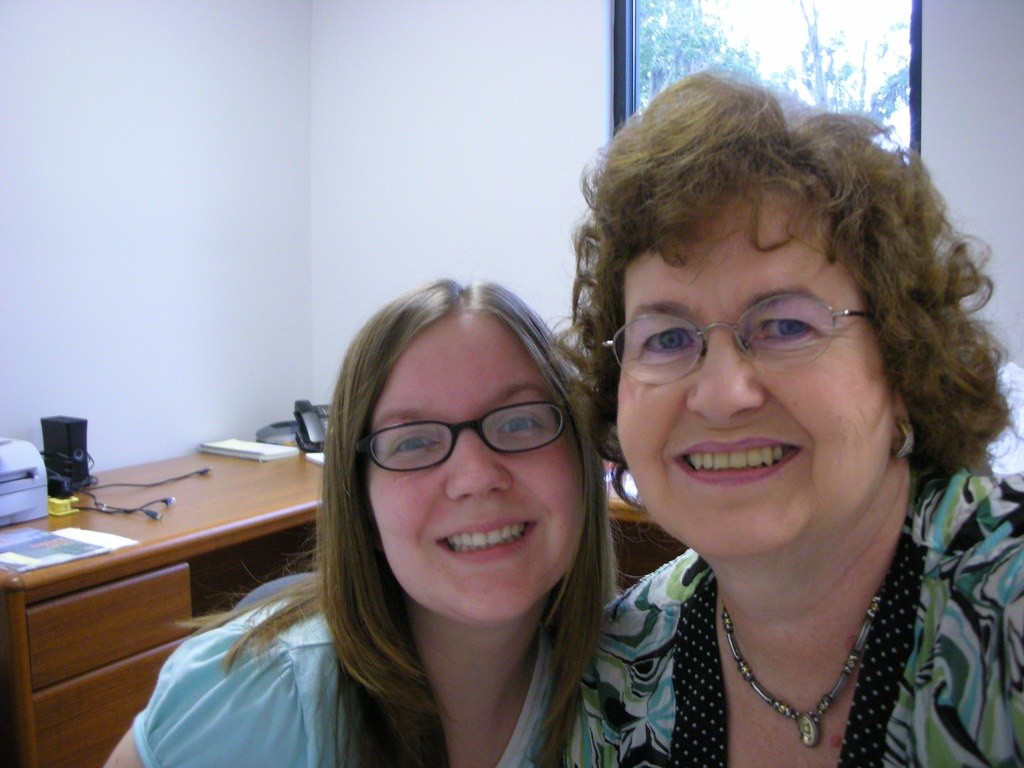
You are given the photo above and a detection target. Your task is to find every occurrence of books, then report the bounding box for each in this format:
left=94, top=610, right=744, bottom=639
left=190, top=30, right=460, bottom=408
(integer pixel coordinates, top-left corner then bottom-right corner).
left=305, top=452, right=325, bottom=466
left=199, top=438, right=299, bottom=462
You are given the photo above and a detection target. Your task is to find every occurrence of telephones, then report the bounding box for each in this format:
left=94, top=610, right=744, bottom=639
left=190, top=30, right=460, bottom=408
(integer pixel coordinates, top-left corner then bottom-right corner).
left=293, top=400, right=331, bottom=453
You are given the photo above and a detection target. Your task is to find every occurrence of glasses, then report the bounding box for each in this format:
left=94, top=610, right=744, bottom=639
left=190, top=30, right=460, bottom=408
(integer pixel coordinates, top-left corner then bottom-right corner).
left=602, top=292, right=874, bottom=384
left=354, top=401, right=570, bottom=472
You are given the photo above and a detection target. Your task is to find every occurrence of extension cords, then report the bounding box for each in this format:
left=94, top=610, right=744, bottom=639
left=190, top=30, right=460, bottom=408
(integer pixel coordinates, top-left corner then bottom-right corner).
left=47, top=495, right=80, bottom=516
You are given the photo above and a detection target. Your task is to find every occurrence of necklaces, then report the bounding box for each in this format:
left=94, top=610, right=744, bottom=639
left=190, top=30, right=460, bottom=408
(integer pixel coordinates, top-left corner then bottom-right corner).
left=720, top=571, right=892, bottom=746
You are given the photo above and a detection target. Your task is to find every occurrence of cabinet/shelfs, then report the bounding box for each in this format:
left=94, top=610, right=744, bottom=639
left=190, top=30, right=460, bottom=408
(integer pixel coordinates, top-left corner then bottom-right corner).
left=0, top=446, right=324, bottom=768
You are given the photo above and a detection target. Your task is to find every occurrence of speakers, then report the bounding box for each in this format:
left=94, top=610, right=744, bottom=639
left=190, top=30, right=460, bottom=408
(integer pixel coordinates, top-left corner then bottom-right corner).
left=41, top=416, right=91, bottom=489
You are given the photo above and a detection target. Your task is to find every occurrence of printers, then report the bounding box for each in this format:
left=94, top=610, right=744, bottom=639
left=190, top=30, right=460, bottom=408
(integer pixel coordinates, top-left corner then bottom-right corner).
left=0, top=439, right=49, bottom=527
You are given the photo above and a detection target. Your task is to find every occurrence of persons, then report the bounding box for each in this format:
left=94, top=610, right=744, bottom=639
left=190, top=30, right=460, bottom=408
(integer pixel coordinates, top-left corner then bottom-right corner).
left=557, top=70, right=1024, bottom=768
left=98, top=277, right=619, bottom=768
